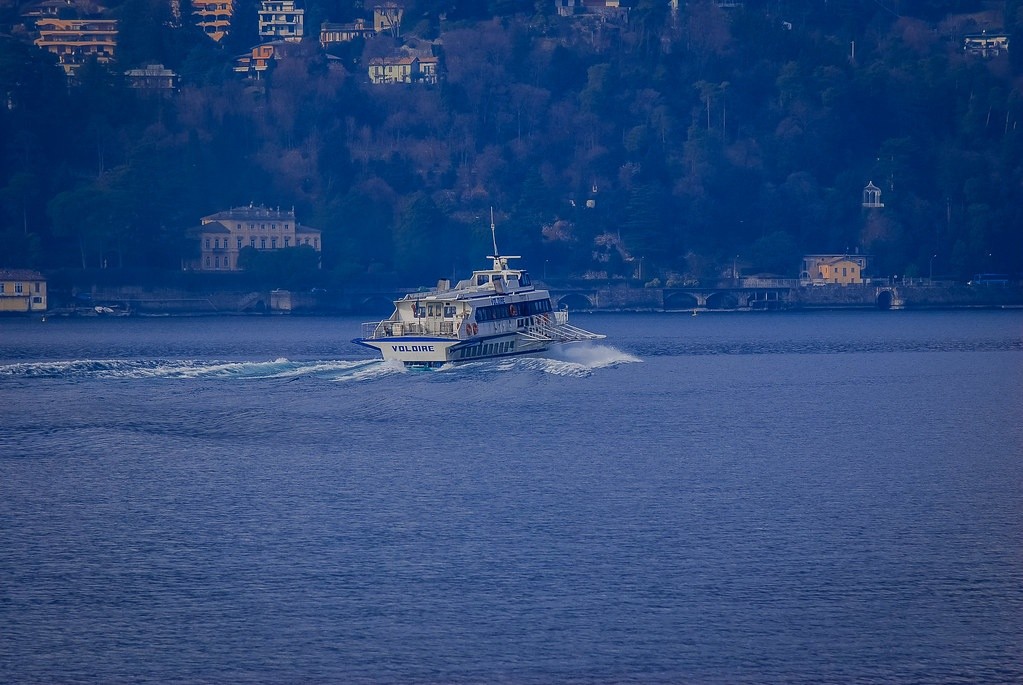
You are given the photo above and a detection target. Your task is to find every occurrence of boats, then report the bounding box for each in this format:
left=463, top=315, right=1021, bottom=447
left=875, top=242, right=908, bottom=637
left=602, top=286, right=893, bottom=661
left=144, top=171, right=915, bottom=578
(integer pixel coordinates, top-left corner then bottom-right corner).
left=349, top=206, right=607, bottom=371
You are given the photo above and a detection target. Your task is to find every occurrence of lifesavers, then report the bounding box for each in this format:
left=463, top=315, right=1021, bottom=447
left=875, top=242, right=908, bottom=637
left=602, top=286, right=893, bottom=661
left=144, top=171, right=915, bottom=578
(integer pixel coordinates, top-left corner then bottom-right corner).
left=509, top=305, right=516, bottom=317
left=544, top=313, right=550, bottom=323
left=465, top=324, right=478, bottom=336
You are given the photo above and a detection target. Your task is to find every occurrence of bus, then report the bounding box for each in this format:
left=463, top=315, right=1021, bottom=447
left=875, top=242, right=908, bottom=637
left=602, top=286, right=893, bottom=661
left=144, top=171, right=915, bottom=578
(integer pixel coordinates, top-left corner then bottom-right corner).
left=972, top=272, right=1008, bottom=286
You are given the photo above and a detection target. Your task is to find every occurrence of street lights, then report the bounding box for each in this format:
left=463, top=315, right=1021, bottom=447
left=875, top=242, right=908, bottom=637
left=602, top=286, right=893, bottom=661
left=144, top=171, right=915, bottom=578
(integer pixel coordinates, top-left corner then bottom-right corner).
left=929, top=255, right=937, bottom=287
